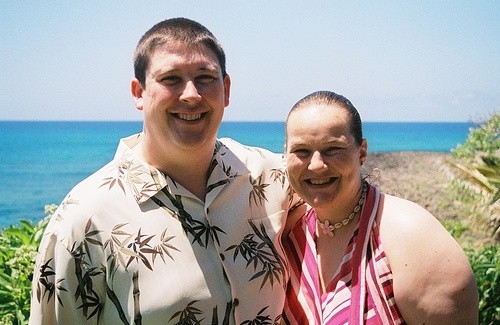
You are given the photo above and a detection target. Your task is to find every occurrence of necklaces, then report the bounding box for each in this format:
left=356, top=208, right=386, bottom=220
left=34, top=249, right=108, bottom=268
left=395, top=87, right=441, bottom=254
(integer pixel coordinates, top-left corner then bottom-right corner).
left=313, top=177, right=367, bottom=238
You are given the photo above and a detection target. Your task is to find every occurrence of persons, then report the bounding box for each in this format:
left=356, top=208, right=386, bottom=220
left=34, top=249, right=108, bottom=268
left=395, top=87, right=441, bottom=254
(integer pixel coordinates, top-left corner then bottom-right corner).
left=26, top=17, right=305, bottom=325
left=277, top=89, right=478, bottom=325
